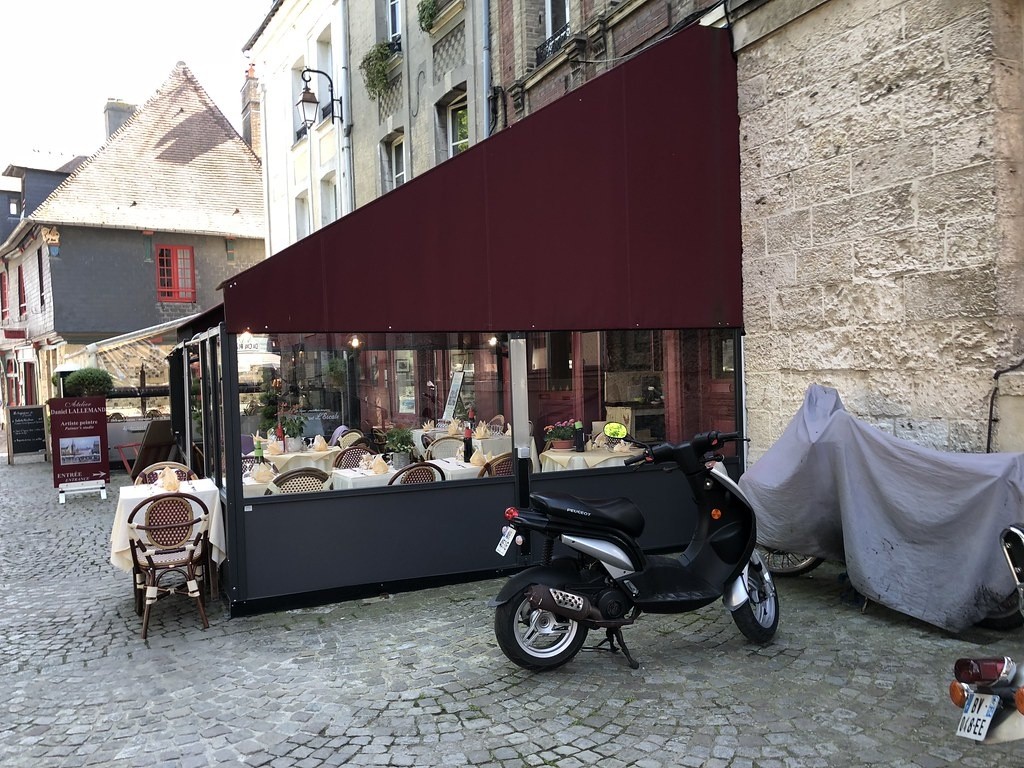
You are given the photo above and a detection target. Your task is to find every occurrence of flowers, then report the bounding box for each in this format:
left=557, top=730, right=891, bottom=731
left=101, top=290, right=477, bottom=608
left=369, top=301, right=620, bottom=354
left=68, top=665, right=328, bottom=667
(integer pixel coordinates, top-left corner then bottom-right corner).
left=544, top=418, right=576, bottom=442
left=375, top=422, right=416, bottom=454
left=277, top=402, right=309, bottom=439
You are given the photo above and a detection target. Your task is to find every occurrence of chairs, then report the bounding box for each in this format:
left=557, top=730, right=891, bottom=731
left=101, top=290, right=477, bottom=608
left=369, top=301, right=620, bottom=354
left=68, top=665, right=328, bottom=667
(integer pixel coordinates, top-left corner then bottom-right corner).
left=144, top=409, right=162, bottom=419
left=108, top=412, right=126, bottom=423
left=133, top=461, right=198, bottom=485
left=127, top=493, right=210, bottom=639
left=240, top=414, right=534, bottom=496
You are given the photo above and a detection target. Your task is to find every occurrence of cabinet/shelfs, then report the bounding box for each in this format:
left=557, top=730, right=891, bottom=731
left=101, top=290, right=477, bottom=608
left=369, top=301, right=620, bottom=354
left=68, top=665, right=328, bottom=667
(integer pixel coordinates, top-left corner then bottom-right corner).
left=604, top=401, right=666, bottom=445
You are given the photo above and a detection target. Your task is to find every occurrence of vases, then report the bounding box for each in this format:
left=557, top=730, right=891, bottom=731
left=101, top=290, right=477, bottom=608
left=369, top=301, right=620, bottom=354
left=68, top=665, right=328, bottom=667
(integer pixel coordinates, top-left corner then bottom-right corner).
left=393, top=451, right=410, bottom=470
left=550, top=439, right=574, bottom=448
left=285, top=436, right=301, bottom=452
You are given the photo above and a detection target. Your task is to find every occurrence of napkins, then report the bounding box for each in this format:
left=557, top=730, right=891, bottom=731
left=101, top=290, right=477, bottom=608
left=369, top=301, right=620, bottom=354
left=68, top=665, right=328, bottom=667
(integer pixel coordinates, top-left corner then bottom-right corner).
left=267, top=439, right=282, bottom=454
left=313, top=435, right=328, bottom=451
left=252, top=462, right=274, bottom=483
left=456, top=443, right=465, bottom=460
left=475, top=420, right=490, bottom=439
left=158, top=466, right=180, bottom=493
left=505, top=422, right=512, bottom=436
left=614, top=440, right=633, bottom=453
left=595, top=432, right=609, bottom=446
left=470, top=449, right=486, bottom=466
left=359, top=452, right=374, bottom=470
left=421, top=420, right=434, bottom=432
left=251, top=430, right=266, bottom=444
left=373, top=455, right=388, bottom=474
left=449, top=417, right=461, bottom=434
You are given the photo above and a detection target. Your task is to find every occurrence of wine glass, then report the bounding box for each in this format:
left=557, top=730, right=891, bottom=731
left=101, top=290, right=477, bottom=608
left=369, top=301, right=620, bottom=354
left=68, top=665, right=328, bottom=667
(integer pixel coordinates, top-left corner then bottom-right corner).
left=360, top=453, right=394, bottom=470
left=488, top=424, right=504, bottom=438
left=435, top=419, right=449, bottom=428
left=253, top=461, right=275, bottom=477
left=299, top=436, right=314, bottom=449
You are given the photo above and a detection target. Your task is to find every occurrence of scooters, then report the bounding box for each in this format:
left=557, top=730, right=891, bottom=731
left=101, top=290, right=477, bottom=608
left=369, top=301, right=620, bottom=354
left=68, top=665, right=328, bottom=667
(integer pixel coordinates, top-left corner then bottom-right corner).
left=949, top=522, right=1024, bottom=746
left=487, top=421, right=780, bottom=671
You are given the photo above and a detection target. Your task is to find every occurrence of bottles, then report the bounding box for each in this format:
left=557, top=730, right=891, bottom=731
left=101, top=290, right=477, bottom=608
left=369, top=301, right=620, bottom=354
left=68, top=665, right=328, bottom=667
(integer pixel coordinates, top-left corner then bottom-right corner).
left=276, top=423, right=285, bottom=452
left=463, top=429, right=472, bottom=462
left=469, top=407, right=475, bottom=432
left=254, top=440, right=264, bottom=465
left=576, top=421, right=585, bottom=452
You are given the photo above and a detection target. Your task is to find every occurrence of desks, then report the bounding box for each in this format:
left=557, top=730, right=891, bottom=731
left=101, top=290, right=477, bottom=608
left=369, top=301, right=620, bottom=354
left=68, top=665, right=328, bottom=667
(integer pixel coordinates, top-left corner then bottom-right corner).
left=539, top=449, right=647, bottom=473
left=110, top=478, right=226, bottom=602
left=333, top=459, right=451, bottom=489
left=246, top=446, right=342, bottom=473
left=213, top=474, right=281, bottom=497
left=411, top=428, right=449, bottom=460
left=424, top=455, right=495, bottom=481
left=435, top=432, right=541, bottom=474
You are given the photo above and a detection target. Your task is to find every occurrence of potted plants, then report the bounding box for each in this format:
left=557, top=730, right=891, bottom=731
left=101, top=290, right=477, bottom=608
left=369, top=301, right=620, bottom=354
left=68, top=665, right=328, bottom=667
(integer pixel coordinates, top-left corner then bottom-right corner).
left=327, top=357, right=348, bottom=386
left=295, top=67, right=344, bottom=130
left=417, top=0, right=451, bottom=36
left=358, top=42, right=402, bottom=101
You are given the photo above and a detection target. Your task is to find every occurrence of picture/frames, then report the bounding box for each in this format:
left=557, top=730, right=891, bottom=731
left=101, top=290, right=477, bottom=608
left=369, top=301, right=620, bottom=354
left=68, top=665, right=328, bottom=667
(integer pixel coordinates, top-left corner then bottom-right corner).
left=396, top=359, right=410, bottom=372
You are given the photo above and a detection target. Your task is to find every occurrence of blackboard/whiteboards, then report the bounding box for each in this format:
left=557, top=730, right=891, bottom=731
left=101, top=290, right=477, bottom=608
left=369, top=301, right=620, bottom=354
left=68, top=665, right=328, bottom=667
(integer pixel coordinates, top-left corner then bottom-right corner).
left=6, top=405, right=50, bottom=457
